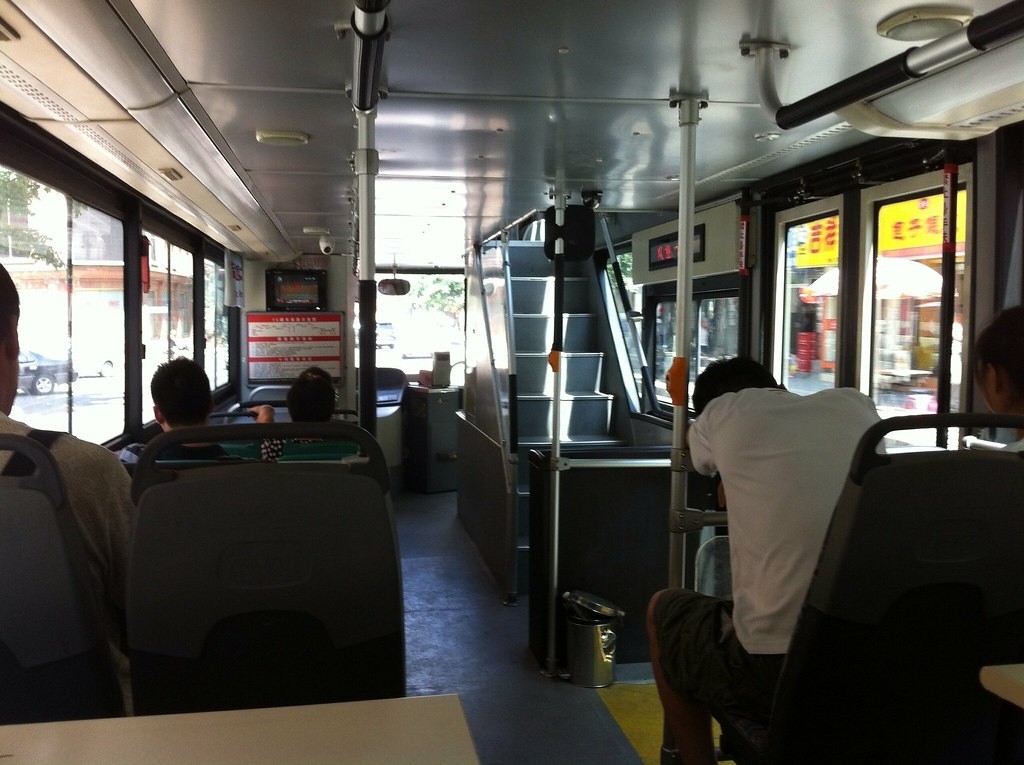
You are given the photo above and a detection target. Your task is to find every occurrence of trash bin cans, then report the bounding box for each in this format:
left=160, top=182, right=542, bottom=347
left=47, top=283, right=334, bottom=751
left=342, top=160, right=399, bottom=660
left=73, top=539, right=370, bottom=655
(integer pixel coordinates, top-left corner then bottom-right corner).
left=562, top=590, right=624, bottom=688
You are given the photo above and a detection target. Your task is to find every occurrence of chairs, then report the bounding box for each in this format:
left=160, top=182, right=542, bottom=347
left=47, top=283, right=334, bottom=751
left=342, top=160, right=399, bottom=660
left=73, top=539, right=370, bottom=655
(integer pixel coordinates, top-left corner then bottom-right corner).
left=0, top=385, right=408, bottom=726
left=709, top=415, right=1024, bottom=765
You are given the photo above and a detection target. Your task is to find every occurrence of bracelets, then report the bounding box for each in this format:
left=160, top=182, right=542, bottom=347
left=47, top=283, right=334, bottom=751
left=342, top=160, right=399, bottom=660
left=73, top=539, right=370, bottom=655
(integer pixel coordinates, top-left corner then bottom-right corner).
left=261, top=405, right=274, bottom=410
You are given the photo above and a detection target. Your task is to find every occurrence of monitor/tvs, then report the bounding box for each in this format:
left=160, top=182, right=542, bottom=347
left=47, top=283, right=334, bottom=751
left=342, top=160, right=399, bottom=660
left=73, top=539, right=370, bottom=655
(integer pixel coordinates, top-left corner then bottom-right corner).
left=265, top=268, right=328, bottom=311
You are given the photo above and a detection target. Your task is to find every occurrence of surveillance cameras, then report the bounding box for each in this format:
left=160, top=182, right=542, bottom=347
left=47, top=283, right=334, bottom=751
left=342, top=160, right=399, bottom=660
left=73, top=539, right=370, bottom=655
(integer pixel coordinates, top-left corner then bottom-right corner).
left=319, top=237, right=335, bottom=255
left=582, top=191, right=603, bottom=209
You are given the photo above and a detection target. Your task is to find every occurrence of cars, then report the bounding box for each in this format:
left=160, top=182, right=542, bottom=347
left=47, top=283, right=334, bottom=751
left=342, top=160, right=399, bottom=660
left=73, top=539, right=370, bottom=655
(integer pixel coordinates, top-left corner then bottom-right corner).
left=376, top=322, right=396, bottom=349
left=53, top=347, right=119, bottom=380
left=16, top=349, right=79, bottom=397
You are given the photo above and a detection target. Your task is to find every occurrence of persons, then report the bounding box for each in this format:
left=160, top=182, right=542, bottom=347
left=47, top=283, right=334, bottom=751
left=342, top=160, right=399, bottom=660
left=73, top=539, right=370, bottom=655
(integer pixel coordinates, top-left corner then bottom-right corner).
left=700, top=317, right=711, bottom=355
left=971, top=301, right=1024, bottom=460
left=0, top=264, right=139, bottom=717
left=120, top=359, right=230, bottom=464
left=647, top=356, right=887, bottom=765
left=246, top=368, right=360, bottom=463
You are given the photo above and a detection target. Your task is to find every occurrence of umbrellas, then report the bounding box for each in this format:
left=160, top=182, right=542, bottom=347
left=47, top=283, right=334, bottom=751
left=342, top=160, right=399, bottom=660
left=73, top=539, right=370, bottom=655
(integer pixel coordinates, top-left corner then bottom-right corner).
left=806, top=254, right=960, bottom=374
